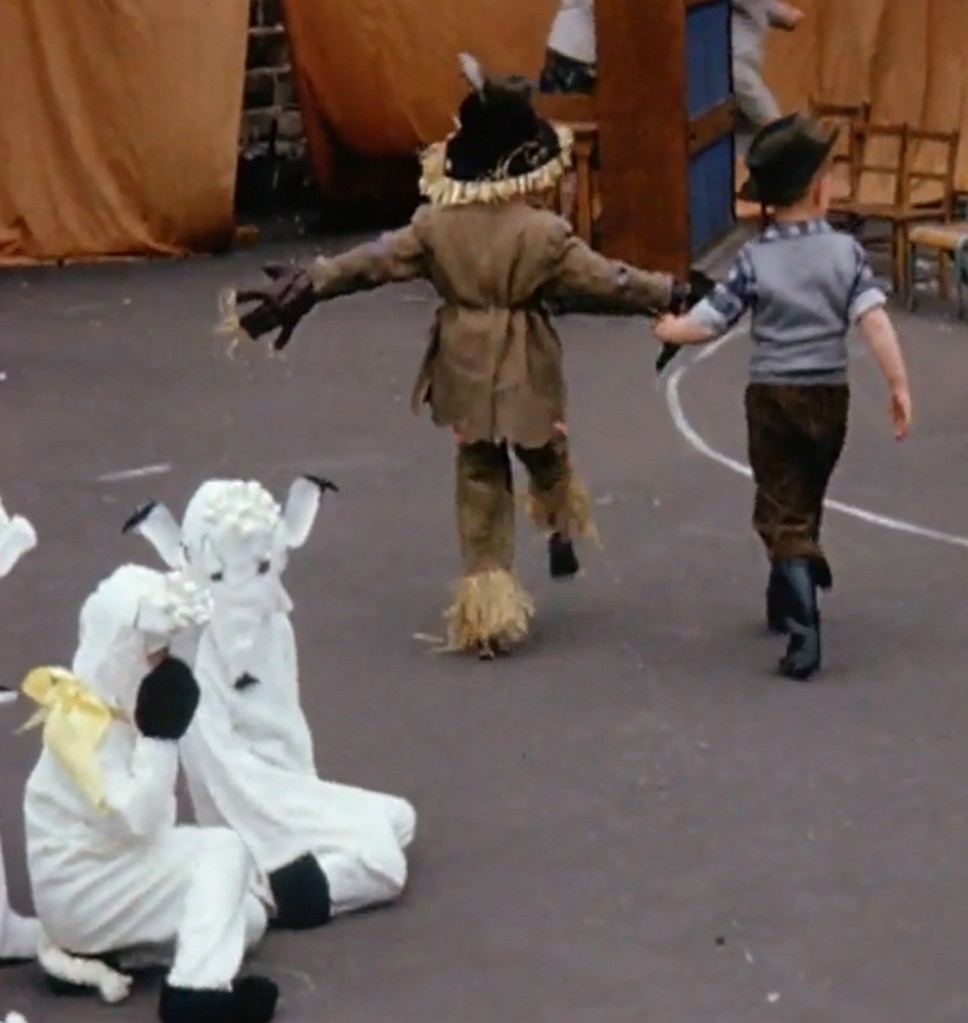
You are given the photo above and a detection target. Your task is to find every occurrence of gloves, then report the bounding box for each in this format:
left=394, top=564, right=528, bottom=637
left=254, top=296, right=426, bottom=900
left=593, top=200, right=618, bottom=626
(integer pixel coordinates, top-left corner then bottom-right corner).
left=669, top=275, right=692, bottom=316
left=133, top=656, right=200, bottom=743
left=213, top=260, right=315, bottom=361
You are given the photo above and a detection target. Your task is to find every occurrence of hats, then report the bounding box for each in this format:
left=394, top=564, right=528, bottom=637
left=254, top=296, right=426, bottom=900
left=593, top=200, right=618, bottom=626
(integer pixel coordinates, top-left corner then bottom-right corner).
left=735, top=111, right=841, bottom=206
left=418, top=52, right=573, bottom=206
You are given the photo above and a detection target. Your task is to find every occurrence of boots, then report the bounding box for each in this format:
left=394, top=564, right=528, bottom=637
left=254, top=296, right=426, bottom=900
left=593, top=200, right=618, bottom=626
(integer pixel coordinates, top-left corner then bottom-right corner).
left=779, top=564, right=822, bottom=680
left=765, top=552, right=791, bottom=635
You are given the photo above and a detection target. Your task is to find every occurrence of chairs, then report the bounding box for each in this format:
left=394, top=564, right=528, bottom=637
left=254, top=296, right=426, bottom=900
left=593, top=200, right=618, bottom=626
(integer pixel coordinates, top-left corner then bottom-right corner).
left=734, top=91, right=967, bottom=320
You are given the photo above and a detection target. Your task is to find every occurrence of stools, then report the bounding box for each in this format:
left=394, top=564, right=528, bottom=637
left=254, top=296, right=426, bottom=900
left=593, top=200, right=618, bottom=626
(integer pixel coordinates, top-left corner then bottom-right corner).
left=550, top=120, right=602, bottom=255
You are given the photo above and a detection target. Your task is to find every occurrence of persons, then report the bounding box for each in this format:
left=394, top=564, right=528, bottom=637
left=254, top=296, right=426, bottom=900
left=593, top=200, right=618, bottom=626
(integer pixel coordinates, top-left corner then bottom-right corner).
left=730, top=0, right=804, bottom=132
left=537, top=0, right=601, bottom=176
left=652, top=114, right=913, bottom=685
left=1, top=473, right=414, bottom=1020
left=223, top=82, right=717, bottom=664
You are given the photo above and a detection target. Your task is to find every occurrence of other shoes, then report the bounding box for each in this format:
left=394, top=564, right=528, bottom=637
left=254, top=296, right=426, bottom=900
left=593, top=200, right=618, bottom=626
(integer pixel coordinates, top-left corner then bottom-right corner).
left=548, top=529, right=581, bottom=578
left=478, top=636, right=508, bottom=660
left=158, top=973, right=279, bottom=1023
left=265, top=851, right=333, bottom=931
